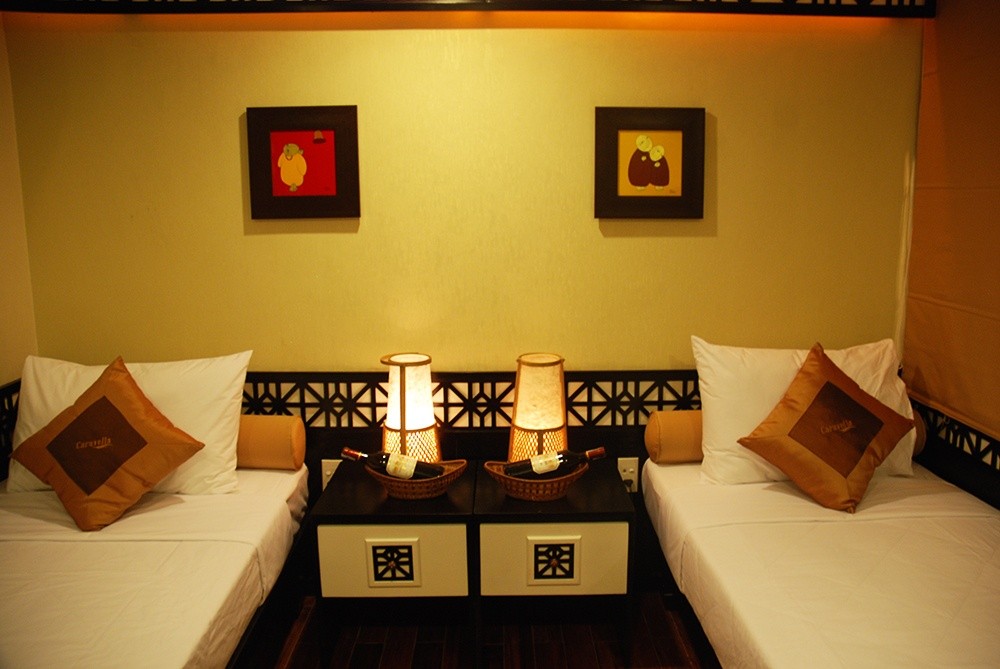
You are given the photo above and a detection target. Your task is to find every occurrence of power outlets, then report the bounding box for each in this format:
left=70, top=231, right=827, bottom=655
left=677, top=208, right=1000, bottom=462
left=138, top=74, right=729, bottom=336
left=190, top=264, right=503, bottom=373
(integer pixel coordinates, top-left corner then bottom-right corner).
left=618, top=458, right=638, bottom=492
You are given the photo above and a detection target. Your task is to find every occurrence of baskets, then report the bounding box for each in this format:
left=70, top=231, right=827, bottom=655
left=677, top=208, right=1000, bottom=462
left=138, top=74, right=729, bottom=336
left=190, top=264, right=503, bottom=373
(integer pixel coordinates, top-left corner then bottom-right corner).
left=365, top=459, right=467, bottom=499
left=485, top=460, right=589, bottom=501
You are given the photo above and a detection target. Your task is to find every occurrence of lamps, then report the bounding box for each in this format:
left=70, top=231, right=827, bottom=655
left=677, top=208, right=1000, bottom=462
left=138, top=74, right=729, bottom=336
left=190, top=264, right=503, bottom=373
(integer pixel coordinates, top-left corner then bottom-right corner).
left=381, top=352, right=443, bottom=464
left=508, top=352, right=569, bottom=462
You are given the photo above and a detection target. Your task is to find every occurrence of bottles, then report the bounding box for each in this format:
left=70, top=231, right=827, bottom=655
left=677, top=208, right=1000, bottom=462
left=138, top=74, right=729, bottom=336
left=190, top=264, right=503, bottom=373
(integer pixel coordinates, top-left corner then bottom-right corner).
left=341, top=447, right=446, bottom=481
left=503, top=447, right=606, bottom=480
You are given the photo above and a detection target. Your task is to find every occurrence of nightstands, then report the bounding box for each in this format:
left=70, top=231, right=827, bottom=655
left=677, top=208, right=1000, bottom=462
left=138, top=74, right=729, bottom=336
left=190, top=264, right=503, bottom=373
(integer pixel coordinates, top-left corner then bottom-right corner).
left=474, top=459, right=637, bottom=669
left=310, top=460, right=475, bottom=669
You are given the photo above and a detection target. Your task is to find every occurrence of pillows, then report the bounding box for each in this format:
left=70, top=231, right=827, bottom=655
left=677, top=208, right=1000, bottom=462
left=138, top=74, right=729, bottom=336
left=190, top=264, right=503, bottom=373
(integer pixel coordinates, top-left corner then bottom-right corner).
left=644, top=411, right=926, bottom=464
left=8, top=355, right=206, bottom=531
left=6, top=355, right=254, bottom=494
left=736, top=342, right=917, bottom=514
left=691, top=335, right=916, bottom=483
left=237, top=415, right=306, bottom=471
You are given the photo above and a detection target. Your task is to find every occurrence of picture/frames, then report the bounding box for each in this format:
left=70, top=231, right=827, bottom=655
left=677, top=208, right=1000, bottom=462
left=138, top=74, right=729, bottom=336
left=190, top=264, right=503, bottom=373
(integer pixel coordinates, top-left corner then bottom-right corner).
left=594, top=107, right=705, bottom=220
left=246, top=105, right=360, bottom=219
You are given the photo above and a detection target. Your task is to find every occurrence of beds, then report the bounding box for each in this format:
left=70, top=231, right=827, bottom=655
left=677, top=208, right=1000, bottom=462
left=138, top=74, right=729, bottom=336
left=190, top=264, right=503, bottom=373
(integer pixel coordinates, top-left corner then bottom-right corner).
left=642, top=457, right=1000, bottom=669
left=0, top=462, right=309, bottom=669
